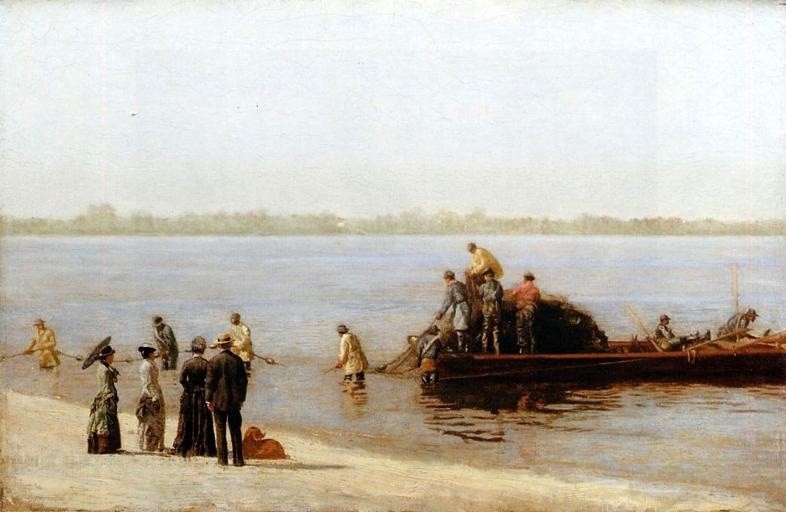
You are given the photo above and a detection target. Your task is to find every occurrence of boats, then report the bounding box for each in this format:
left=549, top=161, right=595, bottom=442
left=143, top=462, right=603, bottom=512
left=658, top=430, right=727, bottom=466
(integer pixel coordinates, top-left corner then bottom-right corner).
left=416, top=326, right=786, bottom=387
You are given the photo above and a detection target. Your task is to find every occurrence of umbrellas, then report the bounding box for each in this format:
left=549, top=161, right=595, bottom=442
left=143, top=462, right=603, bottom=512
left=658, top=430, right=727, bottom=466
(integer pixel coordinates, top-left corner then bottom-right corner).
left=82, top=335, right=120, bottom=375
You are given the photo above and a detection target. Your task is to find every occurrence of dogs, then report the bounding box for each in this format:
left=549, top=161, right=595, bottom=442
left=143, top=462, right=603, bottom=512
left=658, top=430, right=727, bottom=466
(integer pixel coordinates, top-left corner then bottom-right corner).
left=242, top=426, right=291, bottom=459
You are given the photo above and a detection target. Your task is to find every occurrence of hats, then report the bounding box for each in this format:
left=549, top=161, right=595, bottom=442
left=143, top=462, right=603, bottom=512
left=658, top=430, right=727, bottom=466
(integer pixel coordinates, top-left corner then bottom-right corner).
left=152, top=317, right=163, bottom=327
left=444, top=271, right=455, bottom=278
left=138, top=343, right=156, bottom=352
left=337, top=325, right=349, bottom=333
left=99, top=346, right=115, bottom=357
left=231, top=314, right=240, bottom=324
left=481, top=267, right=492, bottom=275
left=468, top=243, right=473, bottom=251
left=213, top=333, right=236, bottom=344
left=660, top=315, right=671, bottom=320
left=749, top=309, right=758, bottom=316
left=524, top=273, right=535, bottom=280
left=32, top=318, right=45, bottom=326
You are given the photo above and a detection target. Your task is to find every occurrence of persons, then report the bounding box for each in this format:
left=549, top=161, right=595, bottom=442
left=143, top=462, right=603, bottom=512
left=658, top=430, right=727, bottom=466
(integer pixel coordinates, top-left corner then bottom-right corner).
left=170, top=336, right=217, bottom=459
left=212, top=313, right=255, bottom=372
left=206, top=330, right=248, bottom=468
left=85, top=346, right=123, bottom=455
left=23, top=319, right=61, bottom=375
left=150, top=315, right=178, bottom=369
left=436, top=241, right=541, bottom=355
left=136, top=347, right=166, bottom=452
left=334, top=323, right=369, bottom=381
